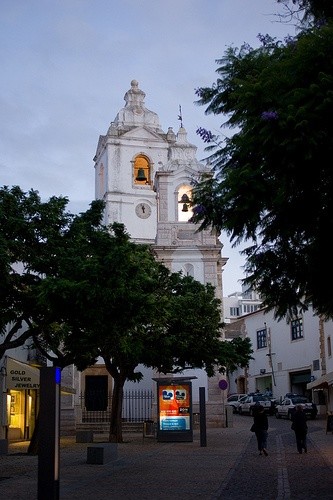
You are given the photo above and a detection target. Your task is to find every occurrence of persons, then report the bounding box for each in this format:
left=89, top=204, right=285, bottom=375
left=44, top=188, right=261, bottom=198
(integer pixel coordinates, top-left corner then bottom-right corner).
left=264, top=387, right=272, bottom=397
left=290, top=404, right=308, bottom=455
left=250, top=405, right=268, bottom=457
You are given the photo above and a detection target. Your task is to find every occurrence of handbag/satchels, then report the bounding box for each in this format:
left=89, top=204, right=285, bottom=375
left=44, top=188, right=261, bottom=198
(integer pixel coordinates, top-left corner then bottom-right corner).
left=249, top=424, right=256, bottom=432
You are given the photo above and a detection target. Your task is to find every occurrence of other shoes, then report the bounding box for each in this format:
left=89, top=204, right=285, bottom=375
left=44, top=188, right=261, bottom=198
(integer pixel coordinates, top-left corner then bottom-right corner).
left=263, top=448, right=270, bottom=456
left=259, top=453, right=263, bottom=457
left=296, top=449, right=307, bottom=455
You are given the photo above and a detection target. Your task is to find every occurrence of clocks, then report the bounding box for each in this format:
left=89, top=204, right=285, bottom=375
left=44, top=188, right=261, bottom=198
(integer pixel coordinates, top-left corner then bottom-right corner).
left=135, top=203, right=151, bottom=219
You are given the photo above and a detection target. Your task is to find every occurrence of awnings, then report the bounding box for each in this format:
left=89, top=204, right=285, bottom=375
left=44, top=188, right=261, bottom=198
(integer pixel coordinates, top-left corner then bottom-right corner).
left=4, top=354, right=40, bottom=390
left=306, top=372, right=333, bottom=390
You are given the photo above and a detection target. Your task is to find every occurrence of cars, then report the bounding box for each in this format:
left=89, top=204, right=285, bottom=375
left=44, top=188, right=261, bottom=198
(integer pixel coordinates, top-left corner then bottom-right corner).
left=237, top=394, right=272, bottom=416
left=274, top=396, right=314, bottom=419
left=227, top=392, right=247, bottom=413
